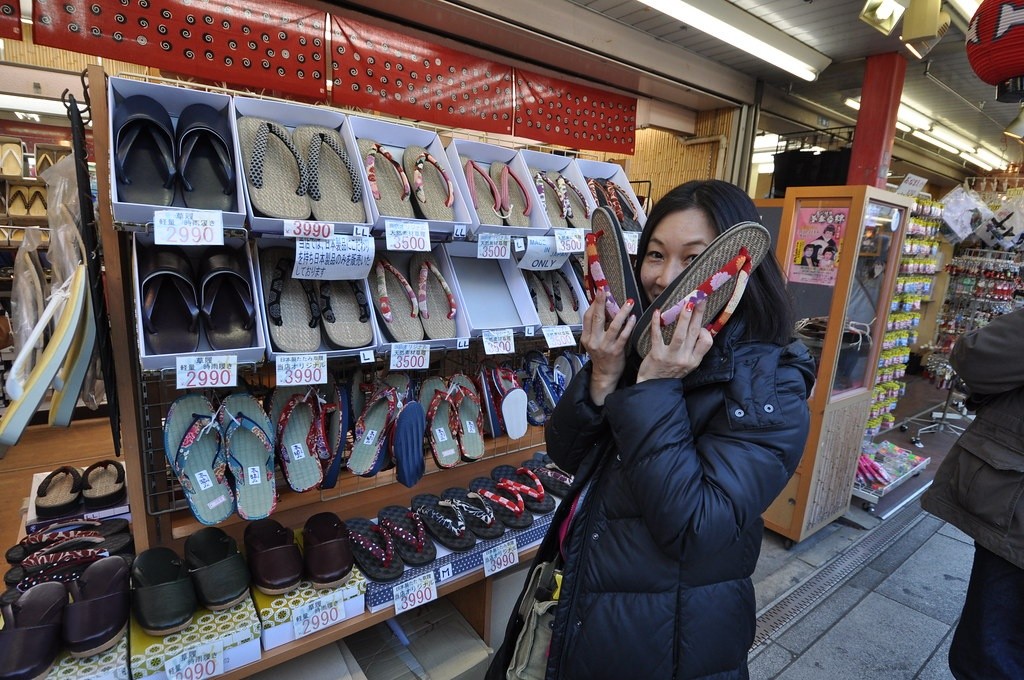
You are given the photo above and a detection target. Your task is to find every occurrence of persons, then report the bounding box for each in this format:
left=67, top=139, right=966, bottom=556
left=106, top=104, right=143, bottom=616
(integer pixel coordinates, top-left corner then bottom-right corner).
left=539, top=180, right=812, bottom=680
left=919, top=306, right=1024, bottom=680
left=800, top=224, right=838, bottom=270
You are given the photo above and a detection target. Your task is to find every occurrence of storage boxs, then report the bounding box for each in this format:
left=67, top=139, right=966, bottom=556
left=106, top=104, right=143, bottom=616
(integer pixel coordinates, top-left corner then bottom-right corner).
left=0, top=75, right=651, bottom=680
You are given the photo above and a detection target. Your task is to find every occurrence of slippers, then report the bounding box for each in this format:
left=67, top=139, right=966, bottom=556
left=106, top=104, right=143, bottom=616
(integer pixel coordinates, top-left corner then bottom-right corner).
left=236, top=115, right=368, bottom=222
left=629, top=218, right=771, bottom=361
left=1, top=346, right=590, bottom=679
left=0, top=144, right=108, bottom=447
left=583, top=206, right=644, bottom=338
left=461, top=156, right=532, bottom=228
left=112, top=94, right=237, bottom=214
left=518, top=256, right=582, bottom=327
left=368, top=250, right=456, bottom=343
left=140, top=239, right=255, bottom=356
left=583, top=175, right=644, bottom=231
left=531, top=168, right=594, bottom=228
left=351, top=138, right=455, bottom=220
left=258, top=245, right=373, bottom=352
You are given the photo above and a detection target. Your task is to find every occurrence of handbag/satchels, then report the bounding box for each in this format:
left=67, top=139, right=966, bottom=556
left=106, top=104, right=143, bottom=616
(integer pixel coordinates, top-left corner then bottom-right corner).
left=505, top=561, right=563, bottom=680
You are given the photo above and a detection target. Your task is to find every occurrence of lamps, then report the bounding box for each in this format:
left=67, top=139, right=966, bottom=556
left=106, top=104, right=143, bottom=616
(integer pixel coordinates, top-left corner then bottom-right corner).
left=1003, top=107, right=1024, bottom=139
left=638, top=0, right=833, bottom=82
left=859, top=0, right=905, bottom=35
left=843, top=95, right=1010, bottom=172
left=899, top=13, right=953, bottom=59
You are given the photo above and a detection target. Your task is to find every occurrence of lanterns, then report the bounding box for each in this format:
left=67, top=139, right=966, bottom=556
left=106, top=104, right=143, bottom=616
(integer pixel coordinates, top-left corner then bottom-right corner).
left=964, top=0, right=1024, bottom=103
